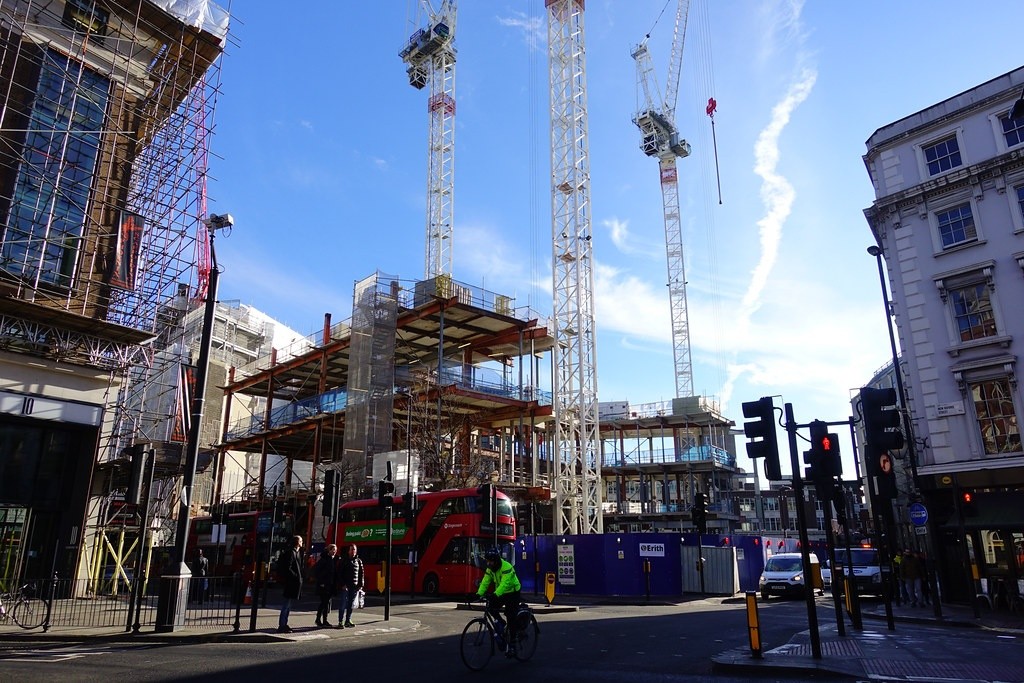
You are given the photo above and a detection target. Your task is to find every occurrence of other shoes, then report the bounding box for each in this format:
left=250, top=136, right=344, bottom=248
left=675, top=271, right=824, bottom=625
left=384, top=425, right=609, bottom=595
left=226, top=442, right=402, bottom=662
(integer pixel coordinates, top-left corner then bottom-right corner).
left=911, top=603, right=917, bottom=608
left=512, top=640, right=522, bottom=655
left=278, top=625, right=292, bottom=633
left=921, top=605, right=926, bottom=608
left=323, top=621, right=332, bottom=627
left=502, top=620, right=506, bottom=627
left=315, top=620, right=322, bottom=627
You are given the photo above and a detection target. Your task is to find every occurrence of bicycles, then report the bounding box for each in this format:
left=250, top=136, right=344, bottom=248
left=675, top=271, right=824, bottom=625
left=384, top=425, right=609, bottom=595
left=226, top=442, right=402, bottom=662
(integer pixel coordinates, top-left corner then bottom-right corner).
left=459, top=596, right=540, bottom=672
left=0, top=584, right=49, bottom=630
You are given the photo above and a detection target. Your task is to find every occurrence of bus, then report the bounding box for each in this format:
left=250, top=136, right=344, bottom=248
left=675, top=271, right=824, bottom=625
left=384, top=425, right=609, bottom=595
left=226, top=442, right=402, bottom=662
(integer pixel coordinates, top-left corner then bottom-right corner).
left=185, top=511, right=296, bottom=592
left=325, top=487, right=517, bottom=597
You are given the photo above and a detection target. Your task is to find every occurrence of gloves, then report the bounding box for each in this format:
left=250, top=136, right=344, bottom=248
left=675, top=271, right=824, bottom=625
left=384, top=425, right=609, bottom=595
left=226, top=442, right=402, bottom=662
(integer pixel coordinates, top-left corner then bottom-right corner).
left=487, top=594, right=498, bottom=601
left=470, top=594, right=480, bottom=602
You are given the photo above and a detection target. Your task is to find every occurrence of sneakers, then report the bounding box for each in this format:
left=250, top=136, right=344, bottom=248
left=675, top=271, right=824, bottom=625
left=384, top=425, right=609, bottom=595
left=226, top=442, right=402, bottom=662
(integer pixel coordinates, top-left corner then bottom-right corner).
left=338, top=621, right=344, bottom=629
left=345, top=620, right=356, bottom=628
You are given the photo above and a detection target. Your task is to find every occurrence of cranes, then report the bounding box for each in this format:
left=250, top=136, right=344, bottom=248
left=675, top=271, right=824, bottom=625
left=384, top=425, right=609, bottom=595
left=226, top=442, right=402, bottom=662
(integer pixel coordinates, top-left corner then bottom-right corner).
left=544, top=0, right=605, bottom=535
left=631, top=1, right=722, bottom=510
left=397, top=0, right=459, bottom=282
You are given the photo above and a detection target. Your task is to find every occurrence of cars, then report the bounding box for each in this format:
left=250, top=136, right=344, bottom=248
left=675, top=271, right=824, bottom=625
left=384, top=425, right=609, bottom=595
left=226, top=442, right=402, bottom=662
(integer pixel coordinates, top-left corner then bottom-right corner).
left=101, top=566, right=134, bottom=592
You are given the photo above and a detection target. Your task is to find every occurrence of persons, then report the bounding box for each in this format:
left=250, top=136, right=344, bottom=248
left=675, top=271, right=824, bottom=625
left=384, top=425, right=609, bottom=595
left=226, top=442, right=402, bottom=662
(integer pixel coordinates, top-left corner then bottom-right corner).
left=336, top=544, right=364, bottom=629
left=276, top=535, right=304, bottom=634
left=311, top=544, right=339, bottom=630
left=891, top=548, right=932, bottom=608
left=475, top=552, right=522, bottom=657
left=190, top=548, right=209, bottom=604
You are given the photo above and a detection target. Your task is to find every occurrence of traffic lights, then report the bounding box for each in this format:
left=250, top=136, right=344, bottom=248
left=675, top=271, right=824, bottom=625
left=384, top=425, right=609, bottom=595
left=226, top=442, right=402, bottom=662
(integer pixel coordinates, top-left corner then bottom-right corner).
left=477, top=484, right=493, bottom=525
left=518, top=503, right=534, bottom=533
left=402, top=492, right=414, bottom=510
left=123, top=444, right=148, bottom=505
left=378, top=480, right=395, bottom=509
left=803, top=433, right=842, bottom=499
left=742, top=397, right=781, bottom=481
left=861, top=387, right=904, bottom=475
left=691, top=492, right=709, bottom=533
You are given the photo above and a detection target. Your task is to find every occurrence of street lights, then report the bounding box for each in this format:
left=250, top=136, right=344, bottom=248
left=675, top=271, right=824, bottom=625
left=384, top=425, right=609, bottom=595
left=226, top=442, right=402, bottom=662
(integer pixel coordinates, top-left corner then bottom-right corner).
left=867, top=246, right=943, bottom=619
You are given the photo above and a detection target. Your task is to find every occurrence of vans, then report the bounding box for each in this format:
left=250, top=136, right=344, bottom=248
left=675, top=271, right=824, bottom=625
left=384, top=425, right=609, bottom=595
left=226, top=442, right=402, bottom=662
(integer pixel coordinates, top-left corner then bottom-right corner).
left=758, top=548, right=882, bottom=601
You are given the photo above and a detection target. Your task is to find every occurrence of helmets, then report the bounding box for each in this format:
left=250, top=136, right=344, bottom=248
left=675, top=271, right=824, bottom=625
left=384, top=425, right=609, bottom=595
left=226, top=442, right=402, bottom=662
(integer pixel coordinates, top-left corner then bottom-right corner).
left=485, top=547, right=502, bottom=573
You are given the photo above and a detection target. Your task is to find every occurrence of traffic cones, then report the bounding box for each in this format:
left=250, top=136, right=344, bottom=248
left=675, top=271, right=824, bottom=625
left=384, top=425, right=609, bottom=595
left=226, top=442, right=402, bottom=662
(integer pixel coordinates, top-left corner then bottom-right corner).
left=244, top=581, right=252, bottom=605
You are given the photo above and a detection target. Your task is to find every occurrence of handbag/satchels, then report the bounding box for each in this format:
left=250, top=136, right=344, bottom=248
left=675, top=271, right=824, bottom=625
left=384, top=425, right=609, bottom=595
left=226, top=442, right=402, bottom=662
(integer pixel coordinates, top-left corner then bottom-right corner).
left=360, top=592, right=364, bottom=609
left=351, top=591, right=360, bottom=609
left=246, top=587, right=252, bottom=597
left=204, top=579, right=208, bottom=590
